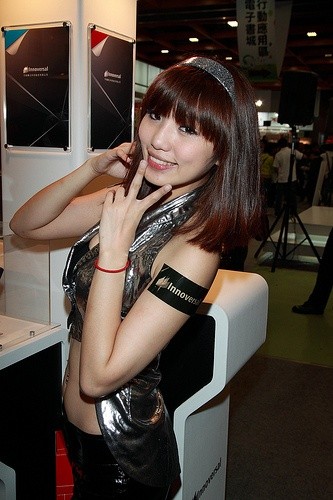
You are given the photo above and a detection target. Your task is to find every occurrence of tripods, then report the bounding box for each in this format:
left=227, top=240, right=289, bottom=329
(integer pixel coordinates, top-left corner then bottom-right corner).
left=255, top=125, right=321, bottom=273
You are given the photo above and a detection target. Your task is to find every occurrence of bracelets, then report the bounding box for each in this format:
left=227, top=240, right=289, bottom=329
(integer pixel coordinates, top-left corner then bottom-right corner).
left=94, top=258, right=130, bottom=273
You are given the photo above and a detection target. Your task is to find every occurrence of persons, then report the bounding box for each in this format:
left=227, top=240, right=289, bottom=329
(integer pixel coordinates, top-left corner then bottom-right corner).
left=313, top=142, right=333, bottom=207
left=10, top=53, right=263, bottom=499
left=305, top=151, right=322, bottom=207
left=292, top=228, right=333, bottom=315
left=272, top=138, right=308, bottom=216
left=258, top=141, right=274, bottom=208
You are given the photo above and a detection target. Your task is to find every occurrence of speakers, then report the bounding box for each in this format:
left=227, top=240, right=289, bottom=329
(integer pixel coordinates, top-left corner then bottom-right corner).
left=278, top=71, right=318, bottom=126
